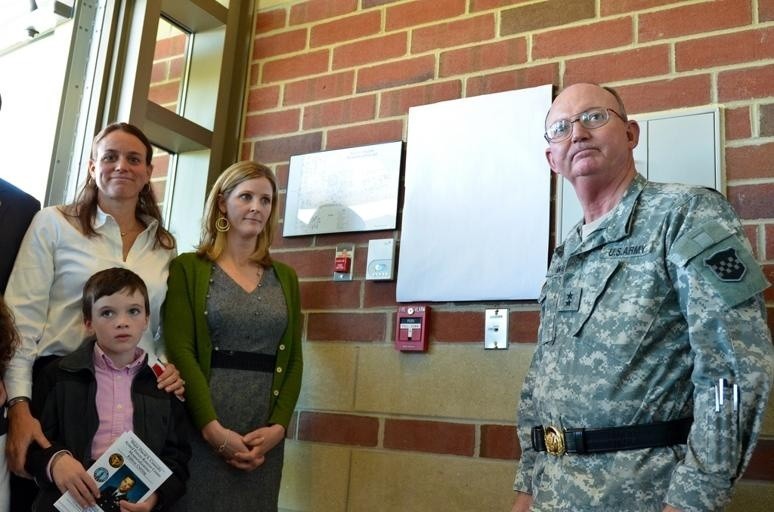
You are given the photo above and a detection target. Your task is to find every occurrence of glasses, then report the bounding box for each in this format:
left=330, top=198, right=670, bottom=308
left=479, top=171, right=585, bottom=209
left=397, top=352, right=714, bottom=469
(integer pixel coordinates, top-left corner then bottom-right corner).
left=543, top=106, right=626, bottom=145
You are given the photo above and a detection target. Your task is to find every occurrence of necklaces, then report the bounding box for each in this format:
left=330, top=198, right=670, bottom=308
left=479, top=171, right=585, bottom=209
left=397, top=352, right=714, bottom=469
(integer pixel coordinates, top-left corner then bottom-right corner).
left=204, top=264, right=263, bottom=356
left=121, top=232, right=128, bottom=236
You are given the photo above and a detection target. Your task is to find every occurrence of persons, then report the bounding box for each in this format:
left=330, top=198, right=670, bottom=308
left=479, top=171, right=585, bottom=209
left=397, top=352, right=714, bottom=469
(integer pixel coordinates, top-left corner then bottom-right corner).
left=4, top=122, right=185, bottom=479
left=512, top=81, right=774, bottom=512
left=161, top=160, right=303, bottom=512
left=8, top=267, right=192, bottom=512
left=0, top=92, right=41, bottom=304
left=0, top=298, right=23, bottom=512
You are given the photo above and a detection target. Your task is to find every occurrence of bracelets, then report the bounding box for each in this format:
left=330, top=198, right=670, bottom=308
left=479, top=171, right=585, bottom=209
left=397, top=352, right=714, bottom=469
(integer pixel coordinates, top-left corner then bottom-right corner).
left=217, top=430, right=230, bottom=454
left=8, top=397, right=27, bottom=407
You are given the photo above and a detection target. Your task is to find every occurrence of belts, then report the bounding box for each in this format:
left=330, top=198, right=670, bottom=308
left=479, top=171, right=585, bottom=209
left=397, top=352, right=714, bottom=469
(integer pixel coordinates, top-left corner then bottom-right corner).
left=530, top=416, right=695, bottom=458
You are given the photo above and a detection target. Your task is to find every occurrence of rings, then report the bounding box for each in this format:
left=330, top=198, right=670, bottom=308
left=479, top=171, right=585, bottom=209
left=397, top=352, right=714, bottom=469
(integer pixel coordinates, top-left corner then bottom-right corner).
left=179, top=379, right=186, bottom=386
